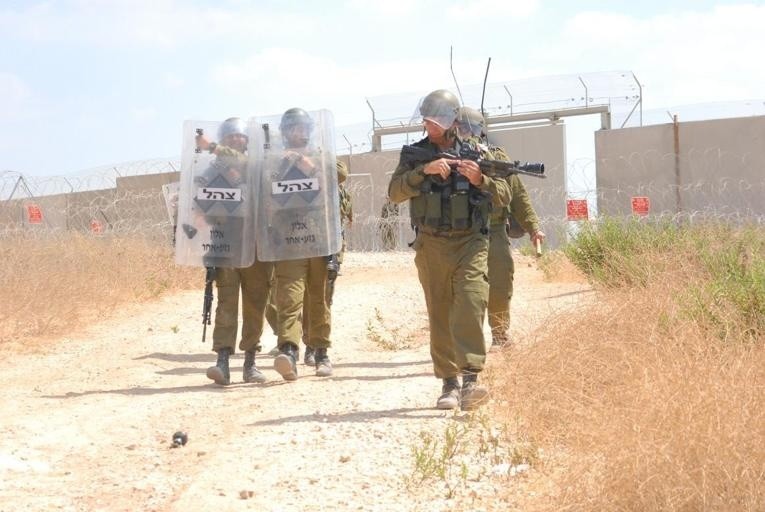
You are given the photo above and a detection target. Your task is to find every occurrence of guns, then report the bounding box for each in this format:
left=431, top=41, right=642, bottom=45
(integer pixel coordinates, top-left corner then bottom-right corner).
left=200, top=231, right=226, bottom=341
left=399, top=144, right=546, bottom=198
left=323, top=255, right=340, bottom=306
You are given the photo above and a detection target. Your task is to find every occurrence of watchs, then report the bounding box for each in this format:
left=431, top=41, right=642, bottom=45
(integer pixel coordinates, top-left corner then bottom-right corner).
left=415, top=163, right=427, bottom=176
left=206, top=141, right=218, bottom=156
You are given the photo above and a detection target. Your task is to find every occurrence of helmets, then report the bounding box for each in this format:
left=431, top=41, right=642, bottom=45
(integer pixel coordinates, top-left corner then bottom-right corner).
left=217, top=117, right=250, bottom=142
left=419, top=89, right=461, bottom=117
left=280, top=107, right=314, bottom=130
left=458, top=107, right=486, bottom=137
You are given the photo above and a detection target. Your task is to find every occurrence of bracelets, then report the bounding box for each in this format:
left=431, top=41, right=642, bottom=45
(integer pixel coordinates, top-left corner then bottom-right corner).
left=294, top=151, right=303, bottom=168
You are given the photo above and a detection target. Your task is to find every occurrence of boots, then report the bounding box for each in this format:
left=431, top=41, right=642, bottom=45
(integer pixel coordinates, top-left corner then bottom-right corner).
left=461, top=374, right=489, bottom=411
left=437, top=376, right=461, bottom=409
left=488, top=330, right=509, bottom=354
left=315, top=348, right=334, bottom=377
left=273, top=342, right=297, bottom=381
left=206, top=350, right=231, bottom=386
left=303, top=345, right=315, bottom=366
left=242, top=350, right=266, bottom=383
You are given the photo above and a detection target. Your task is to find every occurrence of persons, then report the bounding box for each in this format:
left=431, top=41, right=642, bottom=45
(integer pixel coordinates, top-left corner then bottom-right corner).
left=170, top=188, right=179, bottom=243
left=455, top=107, right=544, bottom=353
left=378, top=196, right=399, bottom=248
left=270, top=108, right=347, bottom=380
left=266, top=185, right=351, bottom=367
left=195, top=116, right=275, bottom=384
left=389, top=90, right=513, bottom=411
